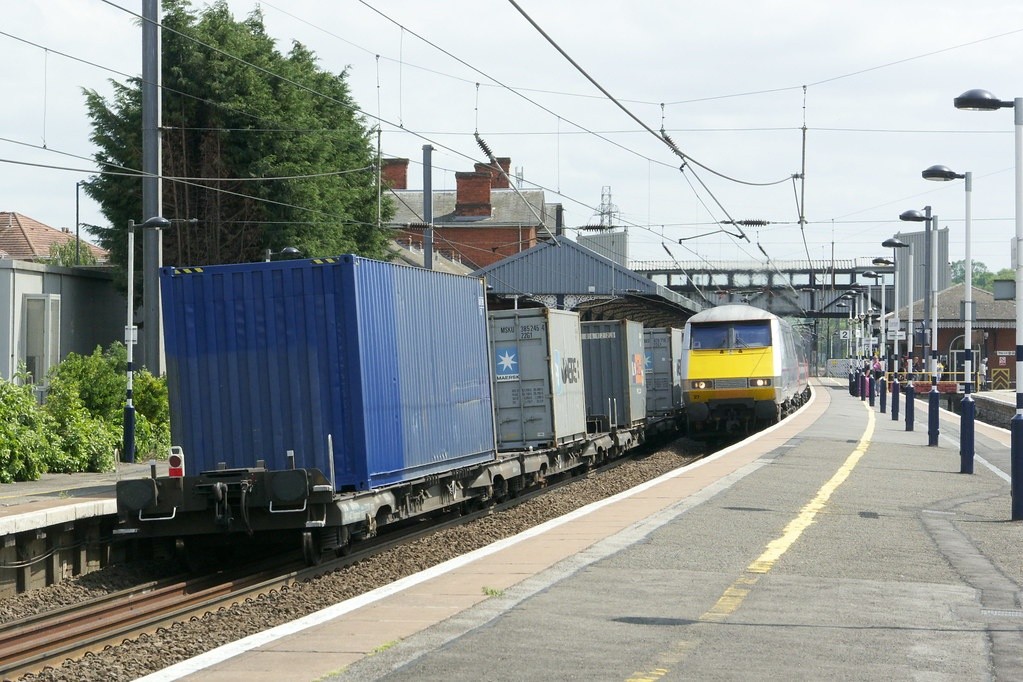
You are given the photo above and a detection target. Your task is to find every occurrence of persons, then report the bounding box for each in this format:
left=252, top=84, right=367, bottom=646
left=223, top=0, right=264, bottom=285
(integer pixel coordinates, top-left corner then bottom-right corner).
left=873, top=357, right=882, bottom=396
left=980, top=361, right=985, bottom=386
left=911, top=354, right=922, bottom=380
left=898, top=363, right=904, bottom=376
left=937, top=361, right=949, bottom=381
left=864, top=360, right=871, bottom=377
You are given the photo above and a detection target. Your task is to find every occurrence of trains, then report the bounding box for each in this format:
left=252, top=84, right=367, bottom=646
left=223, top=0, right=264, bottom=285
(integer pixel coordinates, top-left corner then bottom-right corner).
left=102, top=252, right=685, bottom=560
left=680, top=300, right=812, bottom=433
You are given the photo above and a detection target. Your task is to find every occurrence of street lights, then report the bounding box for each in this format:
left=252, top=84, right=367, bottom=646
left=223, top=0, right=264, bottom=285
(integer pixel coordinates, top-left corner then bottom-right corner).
left=266, top=247, right=300, bottom=264
left=862, top=270, right=886, bottom=414
left=849, top=281, right=876, bottom=407
left=121, top=217, right=172, bottom=464
left=952, top=85, right=1022, bottom=522
left=882, top=238, right=915, bottom=434
left=920, top=164, right=978, bottom=475
left=73, top=179, right=87, bottom=266
left=871, top=255, right=901, bottom=421
left=833, top=288, right=867, bottom=402
left=897, top=205, right=943, bottom=447
